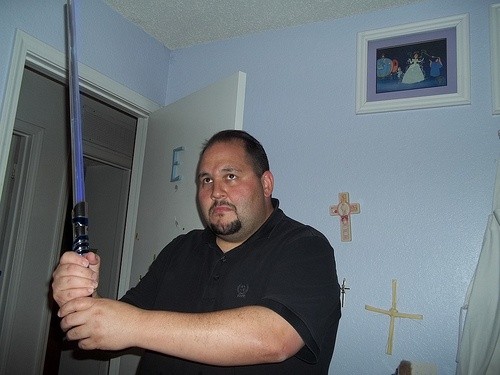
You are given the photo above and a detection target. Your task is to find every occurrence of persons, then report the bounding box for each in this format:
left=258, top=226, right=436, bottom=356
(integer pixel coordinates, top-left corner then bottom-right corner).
left=50, top=130, right=342, bottom=375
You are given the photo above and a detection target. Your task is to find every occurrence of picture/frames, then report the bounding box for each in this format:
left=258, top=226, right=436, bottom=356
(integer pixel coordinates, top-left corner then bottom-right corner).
left=355, top=13, right=471, bottom=115
